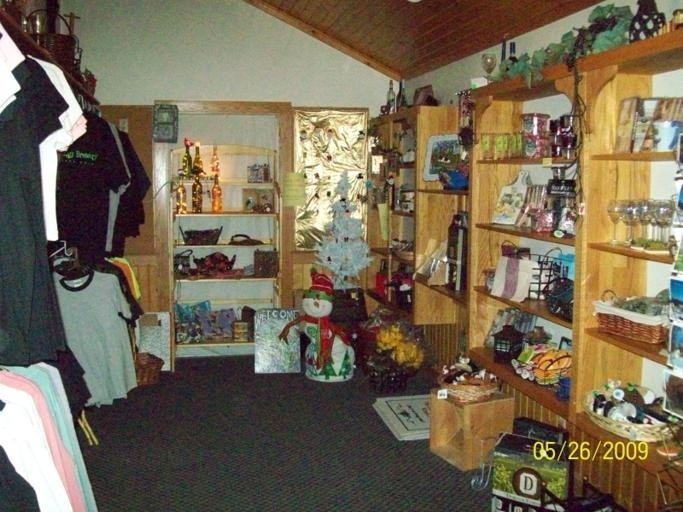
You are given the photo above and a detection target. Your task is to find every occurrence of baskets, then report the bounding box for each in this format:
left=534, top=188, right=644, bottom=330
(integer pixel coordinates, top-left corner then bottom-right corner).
left=178, top=225, right=223, bottom=246
left=597, top=290, right=668, bottom=346
left=514, top=353, right=572, bottom=386
left=128, top=323, right=165, bottom=386
left=436, top=373, right=497, bottom=404
left=583, top=401, right=680, bottom=443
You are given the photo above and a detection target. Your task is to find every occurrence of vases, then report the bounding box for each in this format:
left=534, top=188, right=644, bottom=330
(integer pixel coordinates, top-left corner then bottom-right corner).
left=362, top=361, right=408, bottom=395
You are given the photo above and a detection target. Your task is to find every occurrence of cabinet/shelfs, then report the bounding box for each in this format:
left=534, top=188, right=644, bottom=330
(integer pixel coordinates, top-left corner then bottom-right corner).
left=152, top=101, right=294, bottom=356
left=468, top=58, right=587, bottom=421
left=415, top=106, right=473, bottom=375
left=577, top=57, right=682, bottom=511
left=366, top=109, right=416, bottom=327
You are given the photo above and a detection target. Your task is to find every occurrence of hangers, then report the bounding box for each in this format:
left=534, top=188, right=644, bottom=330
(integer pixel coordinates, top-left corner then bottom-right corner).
left=45, top=239, right=91, bottom=274
left=75, top=92, right=103, bottom=118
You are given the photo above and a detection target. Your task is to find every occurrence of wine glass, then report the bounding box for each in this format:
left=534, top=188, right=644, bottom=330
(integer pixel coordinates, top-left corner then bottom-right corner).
left=481, top=54, right=496, bottom=85
left=178, top=169, right=186, bottom=182
left=607, top=200, right=676, bottom=245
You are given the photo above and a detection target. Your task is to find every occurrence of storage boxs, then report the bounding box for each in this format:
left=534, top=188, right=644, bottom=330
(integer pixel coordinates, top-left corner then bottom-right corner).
left=488, top=431, right=569, bottom=510
left=430, top=376, right=517, bottom=472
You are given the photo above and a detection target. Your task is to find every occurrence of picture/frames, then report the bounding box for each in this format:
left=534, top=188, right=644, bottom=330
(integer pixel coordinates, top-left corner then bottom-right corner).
left=423, top=134, right=463, bottom=180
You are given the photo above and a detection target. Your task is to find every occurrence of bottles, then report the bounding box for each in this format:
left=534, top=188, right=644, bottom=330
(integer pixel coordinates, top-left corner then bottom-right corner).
left=193, top=146, right=202, bottom=168
left=175, top=176, right=186, bottom=214
left=211, top=175, right=222, bottom=212
left=182, top=146, right=192, bottom=180
left=397, top=79, right=407, bottom=107
left=387, top=81, right=396, bottom=114
left=211, top=145, right=220, bottom=179
left=506, top=42, right=518, bottom=69
left=191, top=172, right=202, bottom=213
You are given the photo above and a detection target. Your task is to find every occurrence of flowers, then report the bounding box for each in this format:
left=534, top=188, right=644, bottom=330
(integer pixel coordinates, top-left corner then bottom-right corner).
left=373, top=319, right=425, bottom=371
